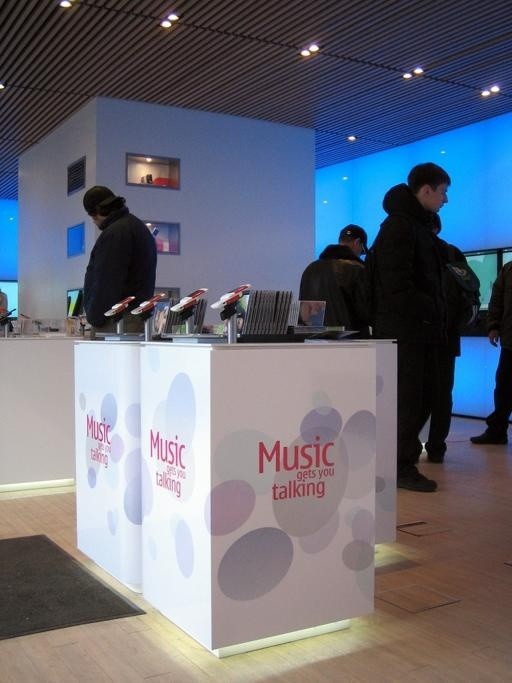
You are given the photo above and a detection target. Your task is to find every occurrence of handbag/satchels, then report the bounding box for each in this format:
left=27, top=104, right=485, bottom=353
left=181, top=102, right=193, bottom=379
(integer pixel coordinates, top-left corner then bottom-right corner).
left=446, top=259, right=481, bottom=324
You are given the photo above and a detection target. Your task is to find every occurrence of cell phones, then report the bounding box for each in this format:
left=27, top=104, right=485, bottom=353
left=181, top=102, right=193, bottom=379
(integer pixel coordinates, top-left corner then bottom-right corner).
left=0, top=308, right=16, bottom=322
left=211, top=284, right=251, bottom=309
left=170, top=287, right=209, bottom=313
left=131, top=293, right=165, bottom=315
left=104, top=296, right=136, bottom=317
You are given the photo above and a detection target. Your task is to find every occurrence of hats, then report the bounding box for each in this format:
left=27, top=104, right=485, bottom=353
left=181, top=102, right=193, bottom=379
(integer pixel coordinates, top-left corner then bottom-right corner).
left=342, top=224, right=369, bottom=254
left=83, top=185, right=119, bottom=208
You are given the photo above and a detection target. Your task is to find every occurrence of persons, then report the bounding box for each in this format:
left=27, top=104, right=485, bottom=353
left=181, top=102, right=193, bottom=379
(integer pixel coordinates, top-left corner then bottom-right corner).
left=416, top=206, right=463, bottom=465
left=81, top=185, right=159, bottom=335
left=470, top=260, right=512, bottom=446
left=362, top=160, right=463, bottom=493
left=298, top=224, right=372, bottom=337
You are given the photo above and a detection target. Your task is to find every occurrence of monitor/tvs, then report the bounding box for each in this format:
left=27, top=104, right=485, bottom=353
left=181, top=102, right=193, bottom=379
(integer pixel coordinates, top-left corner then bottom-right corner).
left=462, top=247, right=512, bottom=311
left=67, top=289, right=82, bottom=316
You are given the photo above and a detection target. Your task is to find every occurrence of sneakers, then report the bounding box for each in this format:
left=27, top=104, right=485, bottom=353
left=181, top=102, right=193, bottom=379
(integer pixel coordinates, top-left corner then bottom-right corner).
left=428, top=453, right=444, bottom=462
left=470, top=428, right=508, bottom=445
left=398, top=467, right=437, bottom=491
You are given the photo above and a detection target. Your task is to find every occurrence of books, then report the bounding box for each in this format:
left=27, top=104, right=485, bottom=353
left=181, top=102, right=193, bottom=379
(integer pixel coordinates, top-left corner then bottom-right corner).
left=223, top=289, right=291, bottom=334
left=151, top=297, right=209, bottom=340
